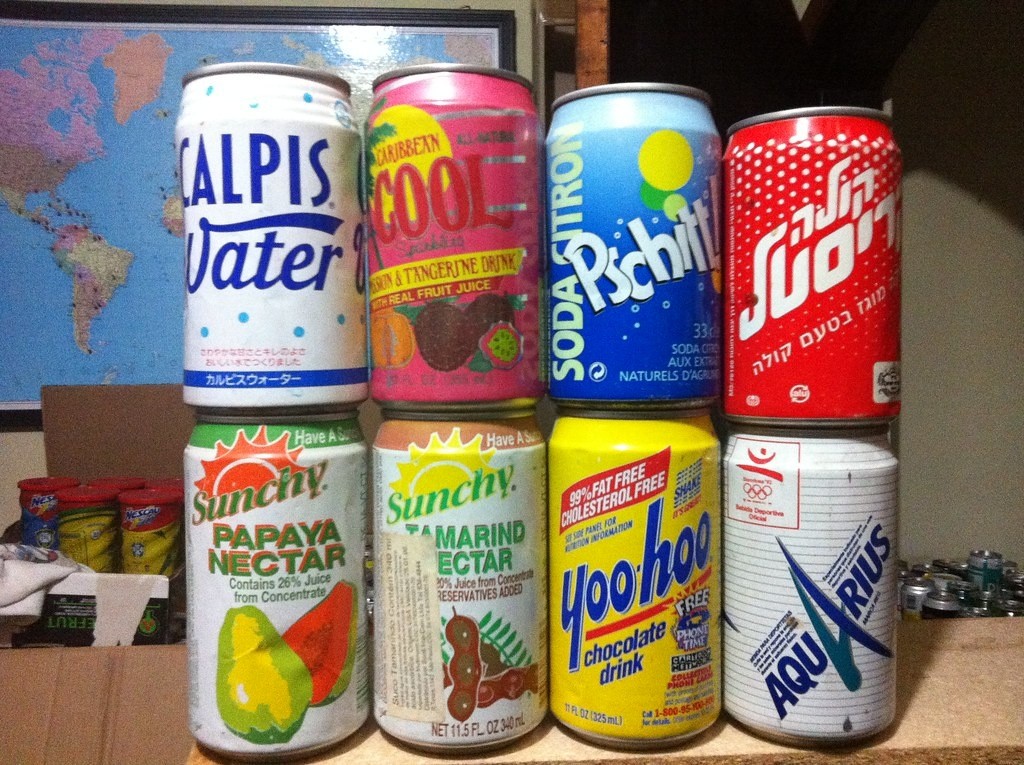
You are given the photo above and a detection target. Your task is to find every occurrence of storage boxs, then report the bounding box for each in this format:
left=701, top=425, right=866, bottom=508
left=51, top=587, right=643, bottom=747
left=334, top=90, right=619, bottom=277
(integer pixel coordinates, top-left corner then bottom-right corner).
left=0, top=383, right=204, bottom=765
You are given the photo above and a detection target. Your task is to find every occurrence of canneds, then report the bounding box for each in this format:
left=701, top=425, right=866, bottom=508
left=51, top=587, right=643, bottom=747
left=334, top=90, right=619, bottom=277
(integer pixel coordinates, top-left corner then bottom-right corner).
left=370, top=407, right=549, bottom=755
left=173, top=61, right=369, bottom=416
left=547, top=406, right=725, bottom=750
left=721, top=105, right=902, bottom=427
left=183, top=411, right=369, bottom=763
left=543, top=80, right=719, bottom=412
left=362, top=61, right=540, bottom=416
left=721, top=426, right=903, bottom=747
left=18, top=474, right=185, bottom=579
left=897, top=547, right=1024, bottom=621
